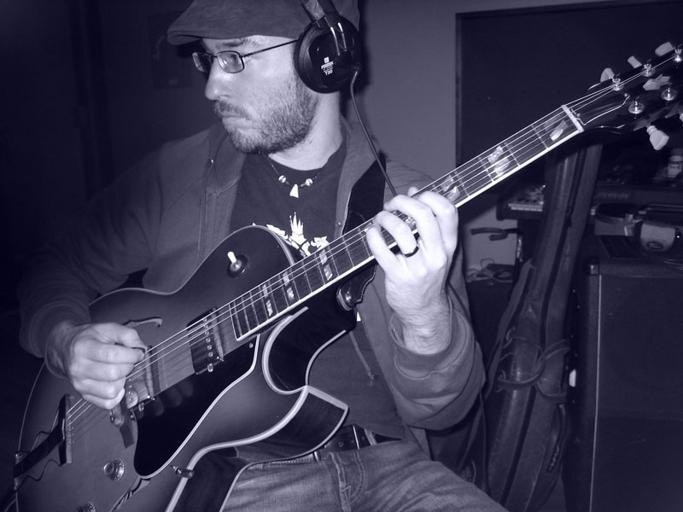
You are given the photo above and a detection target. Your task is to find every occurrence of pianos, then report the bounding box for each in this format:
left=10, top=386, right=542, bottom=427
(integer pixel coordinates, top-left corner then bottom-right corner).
left=496, top=179, right=682, bottom=229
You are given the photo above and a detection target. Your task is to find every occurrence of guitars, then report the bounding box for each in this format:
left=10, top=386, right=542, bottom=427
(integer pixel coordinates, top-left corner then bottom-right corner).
left=12, top=41, right=683, bottom=512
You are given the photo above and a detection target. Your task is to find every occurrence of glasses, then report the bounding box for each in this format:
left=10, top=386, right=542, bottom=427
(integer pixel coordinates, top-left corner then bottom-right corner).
left=191, top=39, right=303, bottom=74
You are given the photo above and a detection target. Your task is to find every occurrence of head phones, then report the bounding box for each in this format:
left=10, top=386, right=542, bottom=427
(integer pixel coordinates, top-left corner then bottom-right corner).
left=294, top=0, right=368, bottom=93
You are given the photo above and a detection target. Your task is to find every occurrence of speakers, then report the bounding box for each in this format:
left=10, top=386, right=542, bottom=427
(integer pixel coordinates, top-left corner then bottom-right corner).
left=574, top=255, right=683, bottom=511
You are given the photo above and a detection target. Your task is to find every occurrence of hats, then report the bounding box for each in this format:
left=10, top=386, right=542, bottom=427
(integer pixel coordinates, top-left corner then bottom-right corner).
left=166, top=0, right=361, bottom=46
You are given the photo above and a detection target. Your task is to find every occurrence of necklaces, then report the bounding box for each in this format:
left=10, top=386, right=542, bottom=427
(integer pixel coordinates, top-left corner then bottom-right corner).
left=264, top=140, right=345, bottom=198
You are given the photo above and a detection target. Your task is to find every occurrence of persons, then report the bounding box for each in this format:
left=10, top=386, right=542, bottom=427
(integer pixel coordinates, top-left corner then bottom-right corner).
left=18, top=0, right=510, bottom=512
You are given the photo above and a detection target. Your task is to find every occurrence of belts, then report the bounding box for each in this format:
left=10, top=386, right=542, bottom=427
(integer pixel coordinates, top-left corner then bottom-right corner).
left=317, top=423, right=402, bottom=454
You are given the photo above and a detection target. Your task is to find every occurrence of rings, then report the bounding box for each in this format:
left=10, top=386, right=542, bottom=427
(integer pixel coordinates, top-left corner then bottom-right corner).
left=402, top=246, right=419, bottom=257
